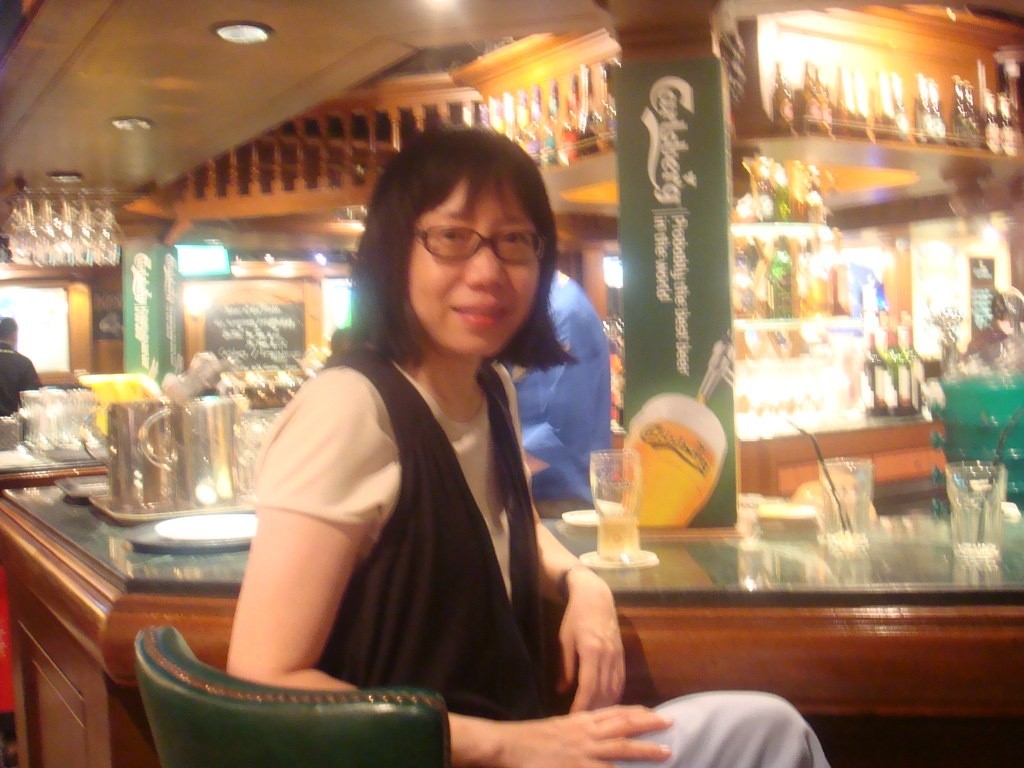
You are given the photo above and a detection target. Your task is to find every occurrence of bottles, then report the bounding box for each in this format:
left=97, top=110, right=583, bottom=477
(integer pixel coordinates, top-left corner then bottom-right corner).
left=189, top=58, right=623, bottom=200
left=886, top=322, right=913, bottom=412
left=729, top=237, right=888, bottom=318
left=742, top=155, right=835, bottom=222
left=768, top=61, right=1023, bottom=156
left=901, top=329, right=925, bottom=411
left=866, top=321, right=888, bottom=414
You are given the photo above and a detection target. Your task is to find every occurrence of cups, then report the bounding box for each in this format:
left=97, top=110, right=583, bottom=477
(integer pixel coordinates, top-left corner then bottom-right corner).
left=818, top=457, right=874, bottom=559
left=589, top=449, right=644, bottom=562
left=948, top=460, right=1007, bottom=560
left=16, top=388, right=95, bottom=450
left=79, top=396, right=242, bottom=512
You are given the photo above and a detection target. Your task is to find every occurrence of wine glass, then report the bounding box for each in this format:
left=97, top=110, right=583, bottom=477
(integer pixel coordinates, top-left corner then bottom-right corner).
left=4, top=192, right=119, bottom=268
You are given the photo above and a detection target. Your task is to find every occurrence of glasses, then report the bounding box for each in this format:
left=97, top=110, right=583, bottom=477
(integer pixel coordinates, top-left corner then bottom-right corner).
left=414, top=224, right=549, bottom=265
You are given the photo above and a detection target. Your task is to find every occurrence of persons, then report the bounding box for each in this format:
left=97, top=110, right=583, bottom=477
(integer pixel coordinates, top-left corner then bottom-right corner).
left=506, top=256, right=611, bottom=519
left=0, top=316, right=40, bottom=416
left=229, top=128, right=834, bottom=767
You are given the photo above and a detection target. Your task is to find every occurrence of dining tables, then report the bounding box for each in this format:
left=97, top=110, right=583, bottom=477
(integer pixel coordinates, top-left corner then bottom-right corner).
left=0, top=431, right=1023, bottom=768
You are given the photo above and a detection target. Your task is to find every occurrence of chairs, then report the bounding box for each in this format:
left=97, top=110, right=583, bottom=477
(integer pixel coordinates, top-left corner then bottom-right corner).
left=132, top=624, right=451, bottom=768
